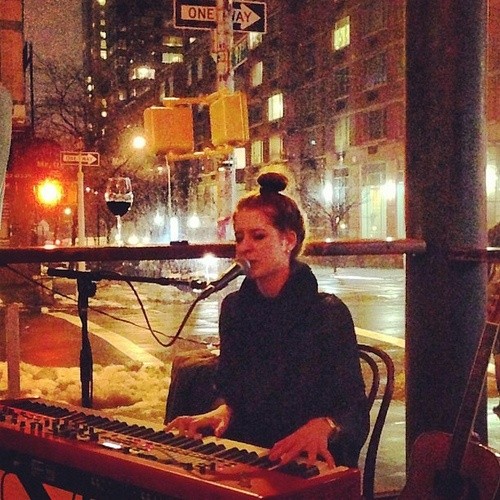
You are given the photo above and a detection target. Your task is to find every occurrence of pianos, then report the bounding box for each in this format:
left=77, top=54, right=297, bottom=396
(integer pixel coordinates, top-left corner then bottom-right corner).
left=0, top=396, right=361, bottom=500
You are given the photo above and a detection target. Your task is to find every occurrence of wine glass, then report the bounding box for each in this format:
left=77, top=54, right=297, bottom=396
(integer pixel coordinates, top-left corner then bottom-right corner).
left=105, top=177, right=133, bottom=247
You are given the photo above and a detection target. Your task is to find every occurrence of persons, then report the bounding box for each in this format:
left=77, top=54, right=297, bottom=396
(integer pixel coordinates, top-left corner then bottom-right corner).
left=487, top=223, right=500, bottom=413
left=164, top=172, right=371, bottom=470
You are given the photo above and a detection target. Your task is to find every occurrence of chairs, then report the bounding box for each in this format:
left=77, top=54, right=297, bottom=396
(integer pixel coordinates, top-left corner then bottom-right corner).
left=338, top=345, right=395, bottom=500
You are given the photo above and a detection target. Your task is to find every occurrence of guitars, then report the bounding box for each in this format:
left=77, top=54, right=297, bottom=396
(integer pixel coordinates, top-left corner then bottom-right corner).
left=398, top=221, right=500, bottom=500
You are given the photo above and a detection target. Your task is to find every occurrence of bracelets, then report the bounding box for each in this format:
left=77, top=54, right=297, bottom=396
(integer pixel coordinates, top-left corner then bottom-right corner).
left=325, top=416, right=338, bottom=431
